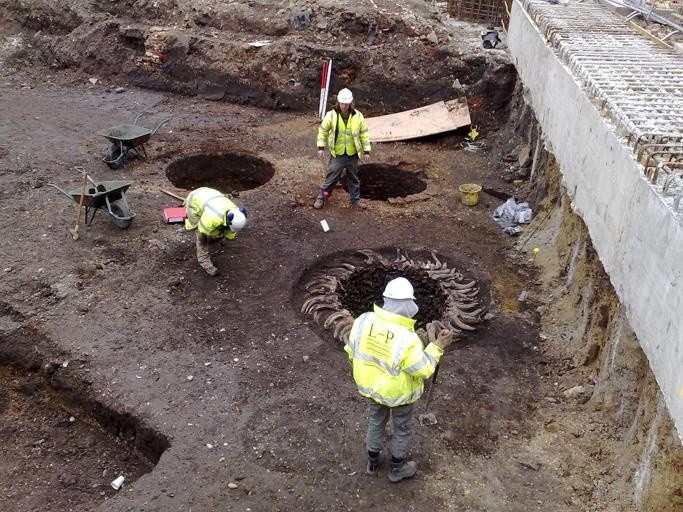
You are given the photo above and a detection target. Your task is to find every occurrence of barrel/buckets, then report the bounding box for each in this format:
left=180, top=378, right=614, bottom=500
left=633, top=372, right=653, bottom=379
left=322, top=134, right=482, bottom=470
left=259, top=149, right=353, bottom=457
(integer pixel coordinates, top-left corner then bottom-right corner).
left=460, top=183, right=482, bottom=206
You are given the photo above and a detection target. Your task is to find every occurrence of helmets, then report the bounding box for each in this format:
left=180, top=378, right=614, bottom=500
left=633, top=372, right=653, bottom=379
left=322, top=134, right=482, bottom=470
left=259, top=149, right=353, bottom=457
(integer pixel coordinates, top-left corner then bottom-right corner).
left=382, top=276, right=416, bottom=300
left=337, top=87, right=354, bottom=104
left=227, top=207, right=247, bottom=231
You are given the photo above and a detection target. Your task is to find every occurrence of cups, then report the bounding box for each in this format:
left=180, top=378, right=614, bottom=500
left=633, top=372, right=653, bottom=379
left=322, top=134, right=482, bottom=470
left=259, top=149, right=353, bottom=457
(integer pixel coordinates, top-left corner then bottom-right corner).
left=110, top=475, right=126, bottom=491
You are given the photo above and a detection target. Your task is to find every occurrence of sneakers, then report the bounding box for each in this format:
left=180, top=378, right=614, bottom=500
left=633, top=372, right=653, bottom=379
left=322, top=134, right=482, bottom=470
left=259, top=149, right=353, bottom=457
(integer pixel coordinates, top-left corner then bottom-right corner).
left=204, top=265, right=220, bottom=275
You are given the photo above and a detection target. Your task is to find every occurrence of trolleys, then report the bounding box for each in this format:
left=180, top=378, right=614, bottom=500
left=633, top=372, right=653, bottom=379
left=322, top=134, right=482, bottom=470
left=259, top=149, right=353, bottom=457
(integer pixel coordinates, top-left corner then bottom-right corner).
left=47, top=180, right=136, bottom=229
left=97, top=113, right=173, bottom=169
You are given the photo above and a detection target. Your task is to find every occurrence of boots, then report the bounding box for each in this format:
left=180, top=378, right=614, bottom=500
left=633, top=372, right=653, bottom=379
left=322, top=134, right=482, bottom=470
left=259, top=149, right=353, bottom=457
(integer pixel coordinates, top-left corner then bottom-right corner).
left=389, top=461, right=417, bottom=483
left=351, top=200, right=368, bottom=209
left=366, top=450, right=384, bottom=475
left=313, top=198, right=324, bottom=209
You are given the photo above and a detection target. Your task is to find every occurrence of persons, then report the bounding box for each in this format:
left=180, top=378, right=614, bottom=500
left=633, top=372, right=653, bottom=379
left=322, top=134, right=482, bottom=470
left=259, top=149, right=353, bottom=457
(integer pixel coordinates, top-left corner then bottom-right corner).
left=185, top=187, right=247, bottom=276
left=344, top=277, right=454, bottom=484
left=313, top=88, right=371, bottom=209
